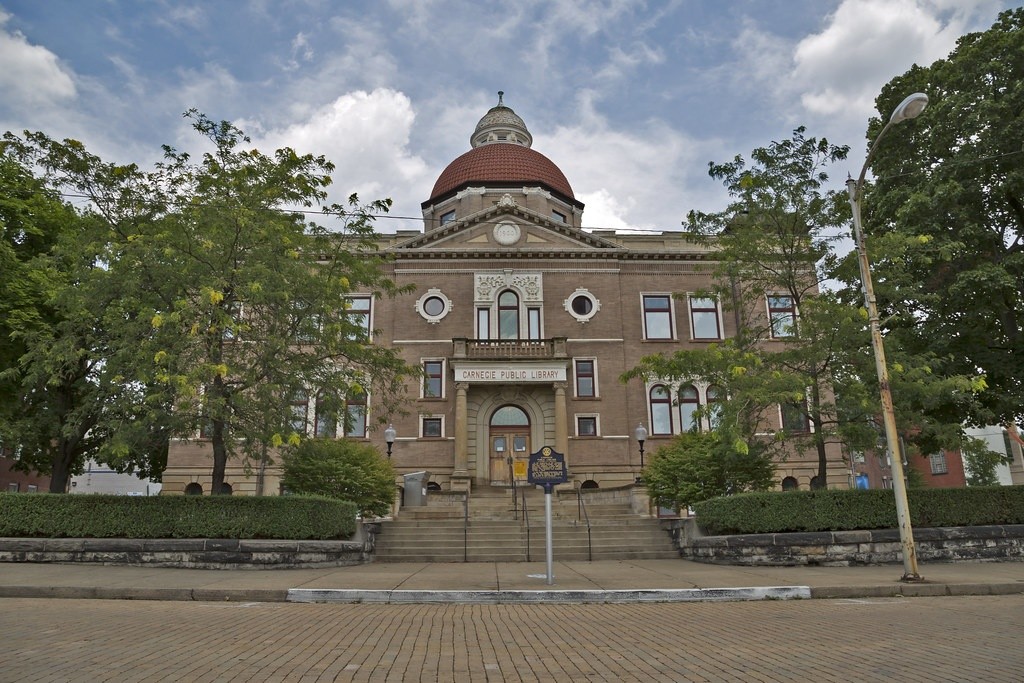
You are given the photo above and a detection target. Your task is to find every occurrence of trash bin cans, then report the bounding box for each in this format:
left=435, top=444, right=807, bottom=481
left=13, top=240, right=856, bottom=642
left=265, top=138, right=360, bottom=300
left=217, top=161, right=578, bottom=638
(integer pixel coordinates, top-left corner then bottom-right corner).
left=403, top=471, right=432, bottom=507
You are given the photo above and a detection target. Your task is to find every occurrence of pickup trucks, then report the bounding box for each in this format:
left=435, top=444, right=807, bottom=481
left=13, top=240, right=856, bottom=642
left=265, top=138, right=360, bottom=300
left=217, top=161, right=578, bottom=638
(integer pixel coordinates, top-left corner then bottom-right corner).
left=847, top=93, right=932, bottom=583
